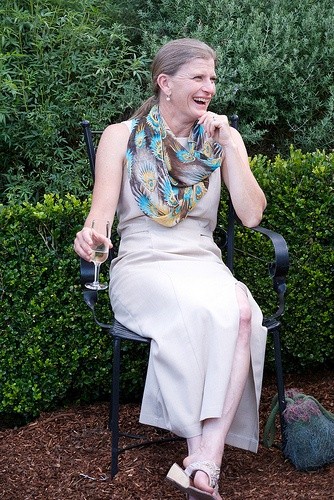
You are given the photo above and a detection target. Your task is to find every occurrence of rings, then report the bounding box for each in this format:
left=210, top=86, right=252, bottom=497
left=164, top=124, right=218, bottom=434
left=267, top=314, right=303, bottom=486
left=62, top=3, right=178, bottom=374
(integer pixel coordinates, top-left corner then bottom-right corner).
left=213, top=116, right=215, bottom=121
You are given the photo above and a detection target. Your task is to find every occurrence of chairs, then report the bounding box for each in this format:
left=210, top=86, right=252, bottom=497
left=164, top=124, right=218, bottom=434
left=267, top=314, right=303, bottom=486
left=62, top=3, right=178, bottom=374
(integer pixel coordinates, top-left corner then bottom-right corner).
left=80, top=115, right=290, bottom=477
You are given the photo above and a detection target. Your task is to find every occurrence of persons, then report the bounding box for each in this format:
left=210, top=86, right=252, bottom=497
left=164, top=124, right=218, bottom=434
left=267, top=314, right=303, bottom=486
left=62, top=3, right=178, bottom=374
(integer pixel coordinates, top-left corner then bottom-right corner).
left=74, top=38, right=267, bottom=500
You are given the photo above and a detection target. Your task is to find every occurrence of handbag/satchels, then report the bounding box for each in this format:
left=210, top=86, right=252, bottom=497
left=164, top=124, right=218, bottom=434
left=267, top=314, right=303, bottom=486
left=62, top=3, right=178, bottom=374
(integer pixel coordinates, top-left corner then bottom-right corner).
left=263, top=388, right=334, bottom=469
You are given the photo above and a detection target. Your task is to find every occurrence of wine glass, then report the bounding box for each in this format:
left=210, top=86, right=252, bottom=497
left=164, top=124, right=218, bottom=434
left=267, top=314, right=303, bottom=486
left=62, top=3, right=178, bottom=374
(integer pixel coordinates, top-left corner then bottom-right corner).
left=84, top=219, right=111, bottom=290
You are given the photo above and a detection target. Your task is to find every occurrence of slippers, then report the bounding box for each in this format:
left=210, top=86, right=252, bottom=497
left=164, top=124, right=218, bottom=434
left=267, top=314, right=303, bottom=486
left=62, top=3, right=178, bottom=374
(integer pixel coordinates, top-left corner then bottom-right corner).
left=166, top=460, right=221, bottom=500
